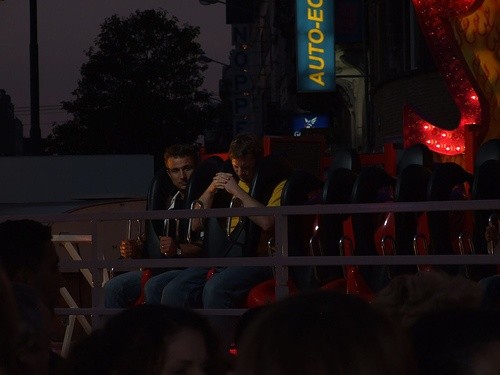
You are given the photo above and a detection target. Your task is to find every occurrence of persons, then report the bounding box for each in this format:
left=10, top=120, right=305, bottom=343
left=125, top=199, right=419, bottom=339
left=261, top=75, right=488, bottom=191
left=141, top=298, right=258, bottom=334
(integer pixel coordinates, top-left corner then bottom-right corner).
left=159, top=133, right=290, bottom=350
left=1, top=218, right=500, bottom=374
left=98, top=136, right=205, bottom=337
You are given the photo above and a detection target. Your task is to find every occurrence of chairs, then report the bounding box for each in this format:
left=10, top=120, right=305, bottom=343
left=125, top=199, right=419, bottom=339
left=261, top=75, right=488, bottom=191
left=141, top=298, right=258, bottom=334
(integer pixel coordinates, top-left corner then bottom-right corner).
left=116, top=137, right=500, bottom=315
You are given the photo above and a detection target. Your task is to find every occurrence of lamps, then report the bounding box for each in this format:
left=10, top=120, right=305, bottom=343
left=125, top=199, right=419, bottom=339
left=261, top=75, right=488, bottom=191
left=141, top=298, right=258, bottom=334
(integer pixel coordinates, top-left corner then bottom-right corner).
left=240, top=88, right=259, bottom=97
left=199, top=54, right=255, bottom=77
left=199, top=0, right=271, bottom=25
left=238, top=40, right=271, bottom=50
